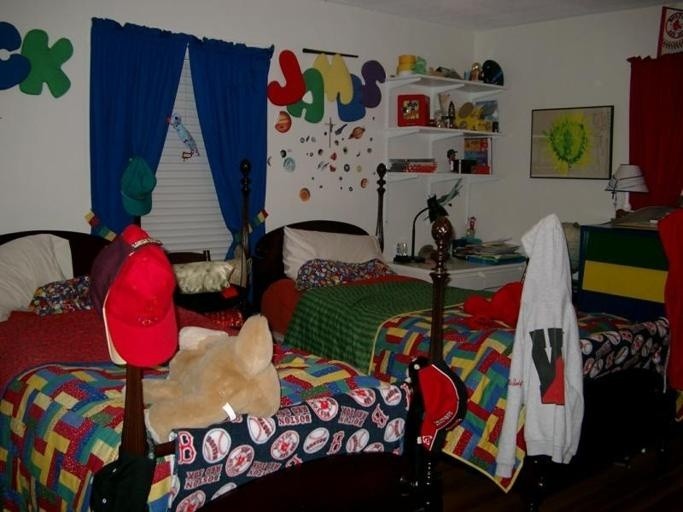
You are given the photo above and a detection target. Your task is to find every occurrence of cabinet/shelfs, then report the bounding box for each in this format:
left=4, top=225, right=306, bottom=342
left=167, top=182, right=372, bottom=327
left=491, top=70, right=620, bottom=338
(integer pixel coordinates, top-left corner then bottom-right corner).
left=385, top=74, right=506, bottom=184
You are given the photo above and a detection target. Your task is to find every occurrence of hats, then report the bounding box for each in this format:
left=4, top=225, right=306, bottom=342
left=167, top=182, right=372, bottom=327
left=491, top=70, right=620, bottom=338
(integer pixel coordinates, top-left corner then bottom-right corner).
left=90, top=225, right=179, bottom=368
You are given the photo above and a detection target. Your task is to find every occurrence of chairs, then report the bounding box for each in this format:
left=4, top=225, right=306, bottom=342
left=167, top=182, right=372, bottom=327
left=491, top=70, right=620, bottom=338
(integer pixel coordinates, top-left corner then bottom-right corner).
left=166, top=250, right=249, bottom=330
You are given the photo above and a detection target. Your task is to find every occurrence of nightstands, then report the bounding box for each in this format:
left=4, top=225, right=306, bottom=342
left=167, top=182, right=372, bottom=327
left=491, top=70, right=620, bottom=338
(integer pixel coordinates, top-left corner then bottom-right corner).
left=387, top=257, right=527, bottom=291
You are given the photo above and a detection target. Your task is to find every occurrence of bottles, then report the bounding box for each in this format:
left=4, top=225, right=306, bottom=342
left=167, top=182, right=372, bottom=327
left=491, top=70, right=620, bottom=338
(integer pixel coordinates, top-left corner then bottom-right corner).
left=466, top=220, right=476, bottom=238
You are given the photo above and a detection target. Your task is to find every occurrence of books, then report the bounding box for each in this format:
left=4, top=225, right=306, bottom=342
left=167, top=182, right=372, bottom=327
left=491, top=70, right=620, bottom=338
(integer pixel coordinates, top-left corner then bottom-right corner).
left=464, top=137, right=492, bottom=175
left=389, top=158, right=436, bottom=173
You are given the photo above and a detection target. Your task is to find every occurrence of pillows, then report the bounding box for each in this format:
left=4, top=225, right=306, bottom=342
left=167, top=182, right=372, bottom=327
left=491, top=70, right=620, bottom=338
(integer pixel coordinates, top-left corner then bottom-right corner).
left=30, top=275, right=93, bottom=313
left=296, top=259, right=385, bottom=291
left=282, top=224, right=385, bottom=281
left=0, top=232, right=74, bottom=323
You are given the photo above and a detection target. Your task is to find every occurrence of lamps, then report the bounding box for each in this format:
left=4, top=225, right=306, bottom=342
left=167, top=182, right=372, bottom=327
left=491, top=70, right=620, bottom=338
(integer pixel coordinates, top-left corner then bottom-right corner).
left=604, top=163, right=649, bottom=213
left=411, top=195, right=448, bottom=263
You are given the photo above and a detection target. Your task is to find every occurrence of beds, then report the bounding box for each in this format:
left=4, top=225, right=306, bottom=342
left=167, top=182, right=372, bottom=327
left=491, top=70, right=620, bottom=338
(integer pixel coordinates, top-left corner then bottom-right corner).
left=240, top=156, right=671, bottom=510
left=0, top=213, right=453, bottom=512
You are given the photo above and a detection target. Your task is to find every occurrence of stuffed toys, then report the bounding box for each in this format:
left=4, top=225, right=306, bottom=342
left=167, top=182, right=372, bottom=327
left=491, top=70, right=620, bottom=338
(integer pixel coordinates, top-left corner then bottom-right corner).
left=450, top=282, right=523, bottom=330
left=142, top=313, right=281, bottom=445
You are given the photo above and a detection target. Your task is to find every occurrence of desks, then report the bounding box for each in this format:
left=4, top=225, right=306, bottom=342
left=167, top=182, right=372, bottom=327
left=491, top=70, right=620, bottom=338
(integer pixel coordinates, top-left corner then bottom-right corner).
left=579, top=223, right=670, bottom=325
